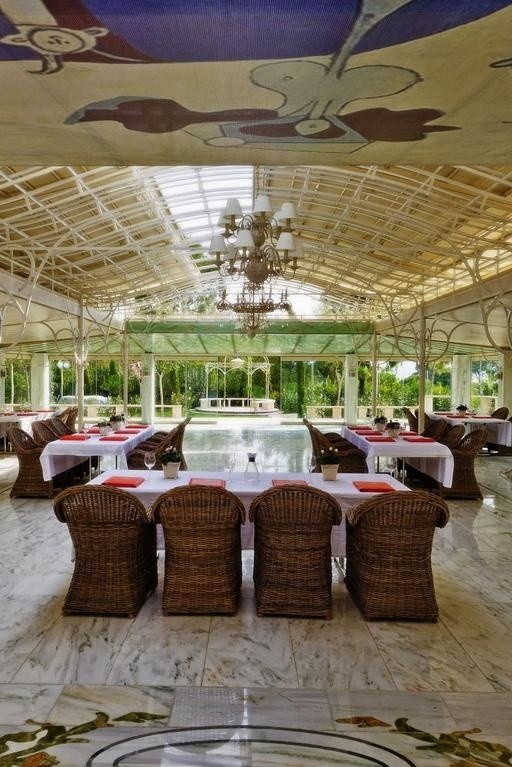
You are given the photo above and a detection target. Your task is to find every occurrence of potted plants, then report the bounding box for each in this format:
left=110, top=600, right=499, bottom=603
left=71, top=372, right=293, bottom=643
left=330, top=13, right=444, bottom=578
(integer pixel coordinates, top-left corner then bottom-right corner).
left=109, top=415, right=125, bottom=430
left=311, top=447, right=342, bottom=481
left=157, top=446, right=183, bottom=478
left=96, top=422, right=111, bottom=436
left=374, top=415, right=388, bottom=432
left=385, top=422, right=401, bottom=439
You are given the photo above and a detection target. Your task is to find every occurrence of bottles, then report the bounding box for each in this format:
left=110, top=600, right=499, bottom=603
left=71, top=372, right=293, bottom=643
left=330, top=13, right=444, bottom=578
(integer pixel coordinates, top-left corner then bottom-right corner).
left=244, top=452, right=259, bottom=482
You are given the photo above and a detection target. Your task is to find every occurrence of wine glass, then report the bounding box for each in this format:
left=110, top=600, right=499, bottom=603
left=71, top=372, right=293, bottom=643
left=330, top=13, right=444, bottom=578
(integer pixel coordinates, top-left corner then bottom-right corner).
left=303, top=456, right=317, bottom=485
left=82, top=423, right=91, bottom=443
left=144, top=452, right=158, bottom=482
left=224, top=453, right=237, bottom=483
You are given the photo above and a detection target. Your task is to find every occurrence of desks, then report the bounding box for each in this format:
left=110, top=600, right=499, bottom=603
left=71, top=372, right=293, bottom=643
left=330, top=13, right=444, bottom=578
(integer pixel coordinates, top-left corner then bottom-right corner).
left=340, top=423, right=455, bottom=490
left=38, top=421, right=154, bottom=482
left=69, top=469, right=415, bottom=581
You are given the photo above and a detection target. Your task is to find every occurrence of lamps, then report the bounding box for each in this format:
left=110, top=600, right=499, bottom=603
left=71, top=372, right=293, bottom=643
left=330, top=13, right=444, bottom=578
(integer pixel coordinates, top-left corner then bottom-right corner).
left=210, top=277, right=295, bottom=336
left=142, top=362, right=150, bottom=378
left=206, top=160, right=308, bottom=291
left=229, top=353, right=243, bottom=368
left=492, top=365, right=503, bottom=380
left=348, top=366, right=356, bottom=377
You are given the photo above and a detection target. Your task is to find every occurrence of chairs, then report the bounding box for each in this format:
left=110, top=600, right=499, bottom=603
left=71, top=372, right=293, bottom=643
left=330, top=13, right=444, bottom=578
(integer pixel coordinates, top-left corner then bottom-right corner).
left=51, top=484, right=158, bottom=619
left=343, top=489, right=452, bottom=624
left=401, top=401, right=511, bottom=502
left=146, top=484, right=247, bottom=618
left=302, top=418, right=368, bottom=475
left=247, top=483, right=345, bottom=623
left=126, top=416, right=191, bottom=471
left=0, top=401, right=97, bottom=500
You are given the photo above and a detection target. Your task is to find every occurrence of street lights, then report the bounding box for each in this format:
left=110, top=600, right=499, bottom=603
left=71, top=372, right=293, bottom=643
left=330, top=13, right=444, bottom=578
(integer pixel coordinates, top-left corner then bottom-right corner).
left=56, top=360, right=71, bottom=397
